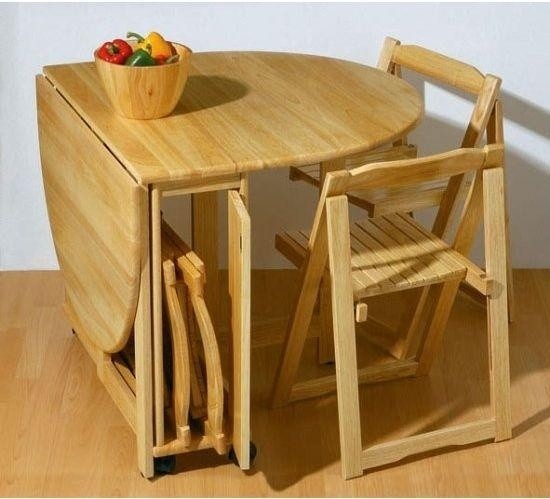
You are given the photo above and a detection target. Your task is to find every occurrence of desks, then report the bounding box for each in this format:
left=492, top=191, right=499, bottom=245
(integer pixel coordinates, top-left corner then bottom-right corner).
left=35, top=51, right=424, bottom=478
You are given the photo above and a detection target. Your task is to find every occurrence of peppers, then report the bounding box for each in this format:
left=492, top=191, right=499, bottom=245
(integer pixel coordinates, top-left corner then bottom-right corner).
left=97, top=30, right=179, bottom=65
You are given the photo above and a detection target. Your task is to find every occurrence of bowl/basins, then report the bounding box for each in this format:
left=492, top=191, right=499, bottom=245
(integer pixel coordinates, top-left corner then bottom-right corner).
left=94, top=39, right=193, bottom=120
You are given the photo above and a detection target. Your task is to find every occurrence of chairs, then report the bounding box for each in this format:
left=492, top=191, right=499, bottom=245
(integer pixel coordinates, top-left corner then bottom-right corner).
left=289, top=35, right=514, bottom=358
left=269, top=143, right=513, bottom=481
